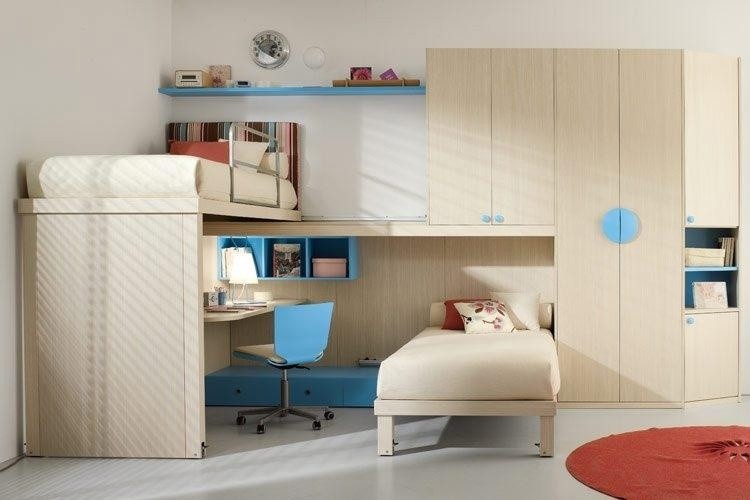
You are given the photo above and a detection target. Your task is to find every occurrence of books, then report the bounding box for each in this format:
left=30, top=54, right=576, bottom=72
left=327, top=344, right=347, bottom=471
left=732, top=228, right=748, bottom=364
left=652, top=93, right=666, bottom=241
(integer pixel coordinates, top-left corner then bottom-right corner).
left=233, top=300, right=268, bottom=308
left=718, top=235, right=734, bottom=267
left=273, top=243, right=300, bottom=277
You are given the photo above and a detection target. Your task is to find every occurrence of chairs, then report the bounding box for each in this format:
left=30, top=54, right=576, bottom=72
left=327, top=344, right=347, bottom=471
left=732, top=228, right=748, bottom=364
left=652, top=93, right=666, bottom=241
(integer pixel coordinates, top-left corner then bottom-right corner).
left=228, top=300, right=342, bottom=434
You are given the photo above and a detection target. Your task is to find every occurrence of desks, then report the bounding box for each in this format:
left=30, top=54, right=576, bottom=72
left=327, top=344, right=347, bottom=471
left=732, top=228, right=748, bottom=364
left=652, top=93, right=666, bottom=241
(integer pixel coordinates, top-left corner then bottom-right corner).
left=203, top=297, right=308, bottom=325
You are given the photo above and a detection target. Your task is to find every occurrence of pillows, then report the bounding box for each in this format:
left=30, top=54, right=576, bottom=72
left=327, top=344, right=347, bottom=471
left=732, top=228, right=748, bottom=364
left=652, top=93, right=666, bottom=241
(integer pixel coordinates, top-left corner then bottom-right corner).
left=452, top=297, right=519, bottom=335
left=438, top=296, right=488, bottom=331
left=489, top=290, right=544, bottom=332
left=217, top=138, right=272, bottom=174
left=166, top=138, right=235, bottom=164
left=255, top=149, right=290, bottom=181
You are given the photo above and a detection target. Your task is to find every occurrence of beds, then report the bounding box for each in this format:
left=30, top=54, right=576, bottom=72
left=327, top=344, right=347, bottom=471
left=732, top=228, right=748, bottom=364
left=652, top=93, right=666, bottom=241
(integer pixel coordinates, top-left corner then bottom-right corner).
left=24, top=121, right=301, bottom=205
left=369, top=293, right=562, bottom=463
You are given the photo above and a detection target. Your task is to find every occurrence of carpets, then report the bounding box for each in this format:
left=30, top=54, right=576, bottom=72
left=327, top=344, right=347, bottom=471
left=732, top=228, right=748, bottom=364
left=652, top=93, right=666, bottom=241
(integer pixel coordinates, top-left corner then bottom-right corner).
left=564, top=424, right=749, bottom=500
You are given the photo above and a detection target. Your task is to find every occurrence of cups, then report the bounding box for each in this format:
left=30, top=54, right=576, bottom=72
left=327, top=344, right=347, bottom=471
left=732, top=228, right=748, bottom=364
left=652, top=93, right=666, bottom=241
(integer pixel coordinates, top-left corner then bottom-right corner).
left=218, top=292, right=226, bottom=305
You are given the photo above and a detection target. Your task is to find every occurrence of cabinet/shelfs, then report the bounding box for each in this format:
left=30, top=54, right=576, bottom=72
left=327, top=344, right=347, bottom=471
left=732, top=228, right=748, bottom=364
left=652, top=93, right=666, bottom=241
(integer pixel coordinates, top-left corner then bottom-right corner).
left=550, top=48, right=685, bottom=412
left=214, top=234, right=360, bottom=283
left=681, top=48, right=743, bottom=230
left=685, top=307, right=742, bottom=409
left=427, top=45, right=556, bottom=229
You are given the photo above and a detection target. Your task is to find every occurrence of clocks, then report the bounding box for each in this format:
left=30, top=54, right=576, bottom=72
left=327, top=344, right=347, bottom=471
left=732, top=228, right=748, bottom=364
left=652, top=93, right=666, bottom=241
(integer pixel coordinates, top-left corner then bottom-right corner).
left=248, top=28, right=292, bottom=71
